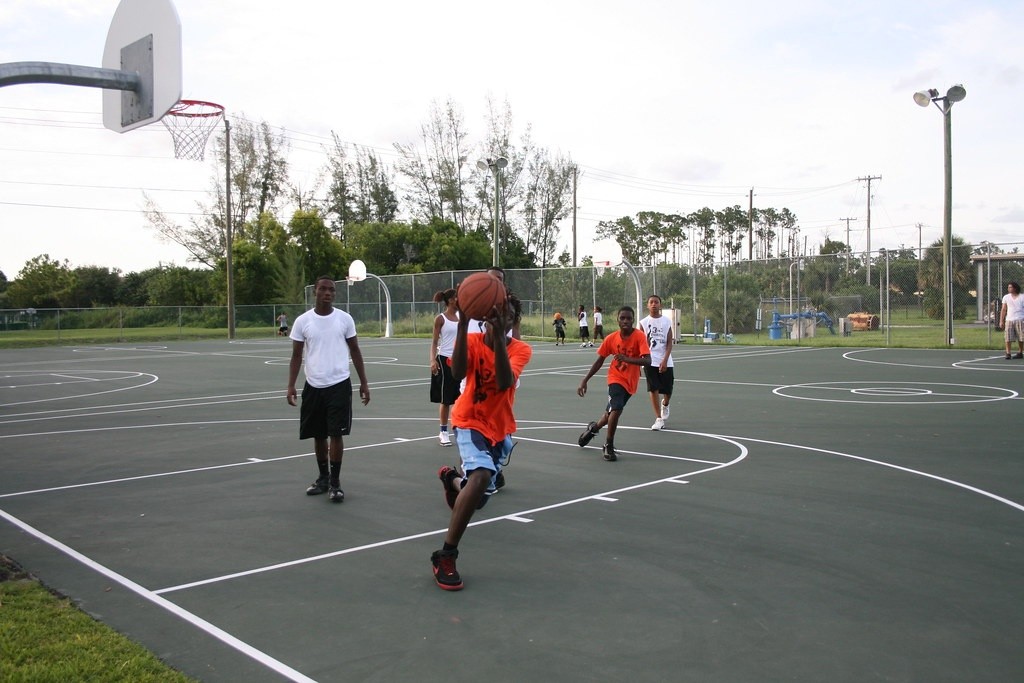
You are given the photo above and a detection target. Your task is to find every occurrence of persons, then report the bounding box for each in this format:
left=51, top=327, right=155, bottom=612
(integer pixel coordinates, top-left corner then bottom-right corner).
left=430, top=265, right=521, bottom=489
left=592, top=306, right=604, bottom=346
left=577, top=306, right=652, bottom=461
left=552, top=312, right=568, bottom=346
left=287, top=275, right=371, bottom=501
left=999, top=281, right=1024, bottom=359
left=636, top=295, right=674, bottom=431
left=276, top=311, right=288, bottom=337
left=578, top=304, right=592, bottom=348
left=430, top=286, right=532, bottom=590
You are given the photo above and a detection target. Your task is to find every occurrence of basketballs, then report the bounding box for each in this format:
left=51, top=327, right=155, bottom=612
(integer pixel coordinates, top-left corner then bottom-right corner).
left=457, top=273, right=507, bottom=320
left=554, top=313, right=562, bottom=319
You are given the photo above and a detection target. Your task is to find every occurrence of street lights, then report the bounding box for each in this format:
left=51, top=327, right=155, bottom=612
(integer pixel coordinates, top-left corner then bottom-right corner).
left=477, top=156, right=509, bottom=267
left=913, top=84, right=967, bottom=347
left=540, top=216, right=563, bottom=275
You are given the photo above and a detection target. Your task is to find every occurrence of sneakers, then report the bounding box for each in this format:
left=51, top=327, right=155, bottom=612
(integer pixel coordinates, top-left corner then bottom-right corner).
left=1005, top=352, right=1011, bottom=360
left=431, top=549, right=464, bottom=590
left=660, top=399, right=670, bottom=420
left=651, top=418, right=665, bottom=431
left=306, top=472, right=344, bottom=502
left=438, top=431, right=453, bottom=446
left=580, top=343, right=587, bottom=348
left=438, top=465, right=462, bottom=510
left=556, top=341, right=558, bottom=345
left=602, top=444, right=617, bottom=461
left=578, top=421, right=599, bottom=447
left=588, top=342, right=595, bottom=347
left=1012, top=352, right=1023, bottom=359
